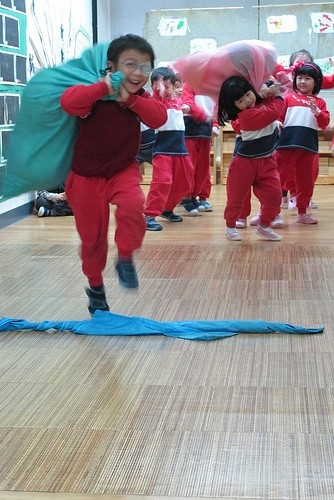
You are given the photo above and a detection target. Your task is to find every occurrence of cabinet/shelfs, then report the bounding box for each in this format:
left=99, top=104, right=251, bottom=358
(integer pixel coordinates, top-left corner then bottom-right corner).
left=141, top=125, right=334, bottom=185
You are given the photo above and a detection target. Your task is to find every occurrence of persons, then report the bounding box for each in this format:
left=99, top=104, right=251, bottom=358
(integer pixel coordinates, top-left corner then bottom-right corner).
left=60, top=35, right=168, bottom=317
left=136, top=49, right=334, bottom=241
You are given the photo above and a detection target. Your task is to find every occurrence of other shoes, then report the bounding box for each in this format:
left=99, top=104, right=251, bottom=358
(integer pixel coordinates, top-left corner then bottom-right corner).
left=309, top=200, right=318, bottom=209
left=280, top=197, right=289, bottom=209
left=288, top=196, right=296, bottom=209
left=200, top=200, right=214, bottom=211
left=37, top=206, right=51, bottom=217
left=180, top=199, right=198, bottom=214
left=197, top=202, right=205, bottom=212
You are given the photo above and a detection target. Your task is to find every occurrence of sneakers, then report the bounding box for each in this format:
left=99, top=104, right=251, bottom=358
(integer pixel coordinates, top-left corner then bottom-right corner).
left=84, top=286, right=110, bottom=317
left=116, top=261, right=140, bottom=289
left=271, top=215, right=284, bottom=228
left=225, top=227, right=242, bottom=241
left=250, top=208, right=262, bottom=226
left=161, top=211, right=183, bottom=222
left=255, top=223, right=282, bottom=241
left=145, top=216, right=163, bottom=231
left=295, top=214, right=319, bottom=224
left=236, top=218, right=247, bottom=227
